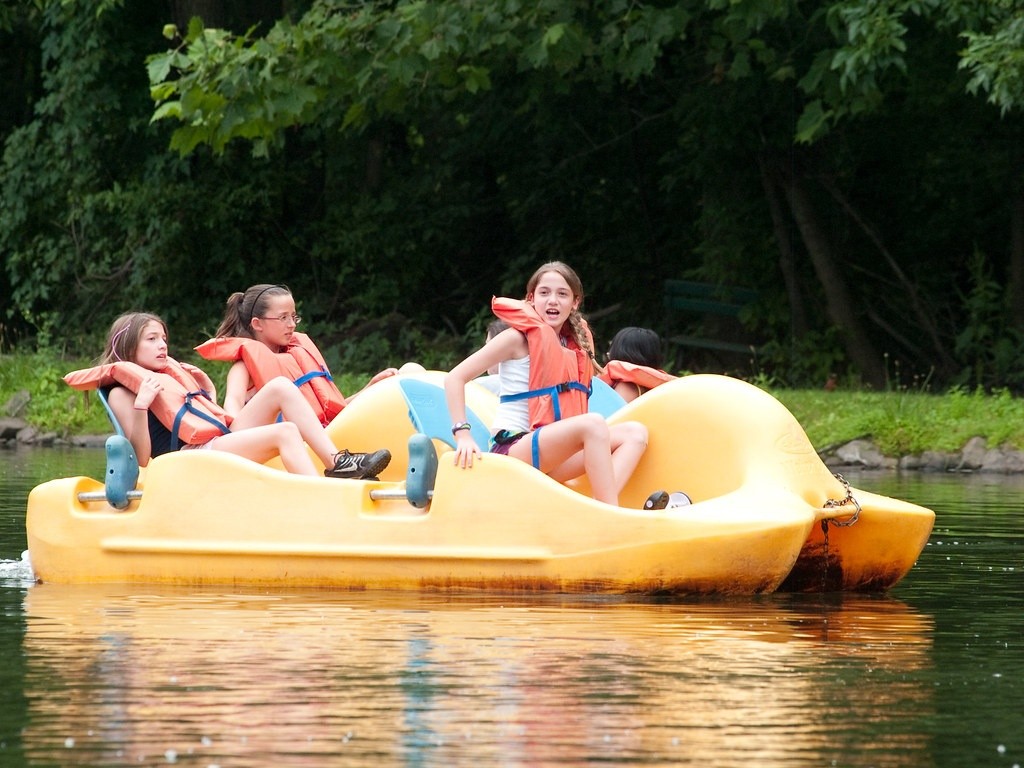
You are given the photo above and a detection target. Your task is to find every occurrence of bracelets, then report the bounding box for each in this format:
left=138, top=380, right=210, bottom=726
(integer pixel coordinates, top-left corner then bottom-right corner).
left=133, top=407, right=149, bottom=411
left=451, top=422, right=471, bottom=436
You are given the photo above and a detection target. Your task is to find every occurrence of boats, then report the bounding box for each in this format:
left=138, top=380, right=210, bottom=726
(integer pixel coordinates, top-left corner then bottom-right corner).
left=26, top=361, right=938, bottom=598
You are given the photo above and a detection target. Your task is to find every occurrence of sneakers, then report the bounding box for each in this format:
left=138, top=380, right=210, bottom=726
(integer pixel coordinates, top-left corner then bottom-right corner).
left=324, top=449, right=391, bottom=481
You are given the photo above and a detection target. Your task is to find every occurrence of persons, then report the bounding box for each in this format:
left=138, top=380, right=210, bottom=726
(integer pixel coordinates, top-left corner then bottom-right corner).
left=192, top=284, right=425, bottom=429
left=485, top=318, right=512, bottom=375
left=443, top=261, right=648, bottom=507
left=595, top=326, right=679, bottom=402
left=63, top=314, right=392, bottom=482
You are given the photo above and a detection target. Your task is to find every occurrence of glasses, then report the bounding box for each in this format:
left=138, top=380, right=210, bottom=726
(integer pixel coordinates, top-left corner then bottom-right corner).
left=258, top=315, right=302, bottom=324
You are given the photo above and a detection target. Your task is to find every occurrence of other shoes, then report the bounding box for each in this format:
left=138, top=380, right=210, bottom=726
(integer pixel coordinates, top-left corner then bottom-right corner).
left=664, top=492, right=692, bottom=509
